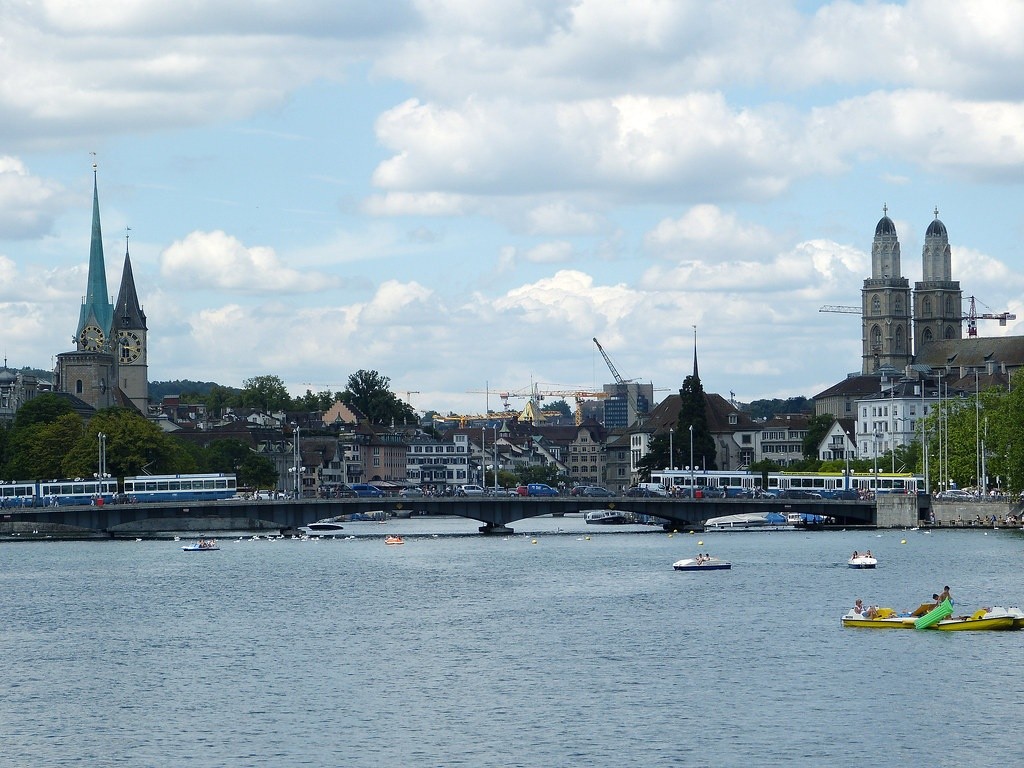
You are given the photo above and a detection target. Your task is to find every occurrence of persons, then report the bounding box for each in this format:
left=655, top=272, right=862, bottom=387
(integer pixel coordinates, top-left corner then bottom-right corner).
left=990, top=489, right=995, bottom=499
left=933, top=586, right=954, bottom=620
left=696, top=553, right=710, bottom=565
left=825, top=518, right=832, bottom=524
left=385, top=535, right=401, bottom=541
left=813, top=517, right=817, bottom=524
left=643, top=484, right=650, bottom=498
left=197, top=538, right=215, bottom=548
left=482, top=486, right=489, bottom=493
left=957, top=514, right=1017, bottom=525
left=855, top=599, right=882, bottom=619
left=916, top=487, right=918, bottom=494
left=933, top=489, right=937, bottom=501
left=89, top=492, right=137, bottom=506
left=672, top=485, right=675, bottom=497
left=858, top=487, right=875, bottom=501
left=416, top=484, right=467, bottom=498
left=239, top=482, right=351, bottom=500
left=803, top=517, right=807, bottom=524
left=723, top=485, right=728, bottom=498
left=853, top=550, right=858, bottom=559
left=0, top=492, right=59, bottom=508
left=753, top=486, right=756, bottom=499
left=865, top=550, right=872, bottom=558
left=930, top=510, right=935, bottom=517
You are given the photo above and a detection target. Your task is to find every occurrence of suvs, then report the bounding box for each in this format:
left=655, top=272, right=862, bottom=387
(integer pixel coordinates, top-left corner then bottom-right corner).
left=583, top=487, right=616, bottom=498
left=779, top=489, right=823, bottom=500
left=570, top=485, right=588, bottom=497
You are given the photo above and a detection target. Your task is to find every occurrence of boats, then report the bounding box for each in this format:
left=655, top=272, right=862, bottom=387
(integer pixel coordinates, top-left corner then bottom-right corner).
left=848, top=555, right=878, bottom=569
left=703, top=513, right=835, bottom=532
left=579, top=511, right=671, bottom=524
left=385, top=536, right=405, bottom=545
left=673, top=557, right=732, bottom=570
left=307, top=523, right=344, bottom=530
left=180, top=541, right=220, bottom=551
left=840, top=605, right=1024, bottom=632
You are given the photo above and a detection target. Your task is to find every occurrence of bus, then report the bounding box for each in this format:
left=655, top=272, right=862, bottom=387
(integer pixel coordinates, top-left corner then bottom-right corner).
left=0, top=473, right=238, bottom=510
left=650, top=469, right=926, bottom=494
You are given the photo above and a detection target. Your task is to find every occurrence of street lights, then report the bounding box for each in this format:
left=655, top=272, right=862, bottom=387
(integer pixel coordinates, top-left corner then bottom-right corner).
left=102, top=434, right=106, bottom=472
left=292, top=428, right=297, bottom=500
left=481, top=426, right=486, bottom=489
left=873, top=428, right=878, bottom=495
left=689, top=425, right=694, bottom=499
left=97, top=432, right=103, bottom=499
left=669, top=428, right=674, bottom=470
left=296, top=427, right=302, bottom=500
left=846, top=430, right=850, bottom=476
left=492, top=423, right=498, bottom=497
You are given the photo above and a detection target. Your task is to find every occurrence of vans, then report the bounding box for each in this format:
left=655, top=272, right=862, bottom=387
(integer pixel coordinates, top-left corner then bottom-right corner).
left=528, top=483, right=559, bottom=498
left=319, top=483, right=359, bottom=499
left=351, top=483, right=386, bottom=499
left=638, top=483, right=672, bottom=498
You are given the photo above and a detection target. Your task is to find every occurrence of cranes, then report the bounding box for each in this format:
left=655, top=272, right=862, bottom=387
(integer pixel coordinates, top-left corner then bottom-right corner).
left=432, top=382, right=610, bottom=427
left=592, top=337, right=654, bottom=420
left=817, top=294, right=1016, bottom=339
left=396, top=391, right=420, bottom=405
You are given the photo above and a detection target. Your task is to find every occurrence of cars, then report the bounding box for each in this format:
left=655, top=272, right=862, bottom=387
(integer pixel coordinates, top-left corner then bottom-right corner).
left=462, top=485, right=483, bottom=496
left=516, top=486, right=528, bottom=497
left=833, top=490, right=861, bottom=500
left=398, top=487, right=431, bottom=498
left=889, top=489, right=913, bottom=495
left=736, top=488, right=777, bottom=500
left=488, top=486, right=517, bottom=497
left=699, top=486, right=725, bottom=498
left=254, top=490, right=286, bottom=501
left=622, top=487, right=660, bottom=498
left=936, top=489, right=976, bottom=499
